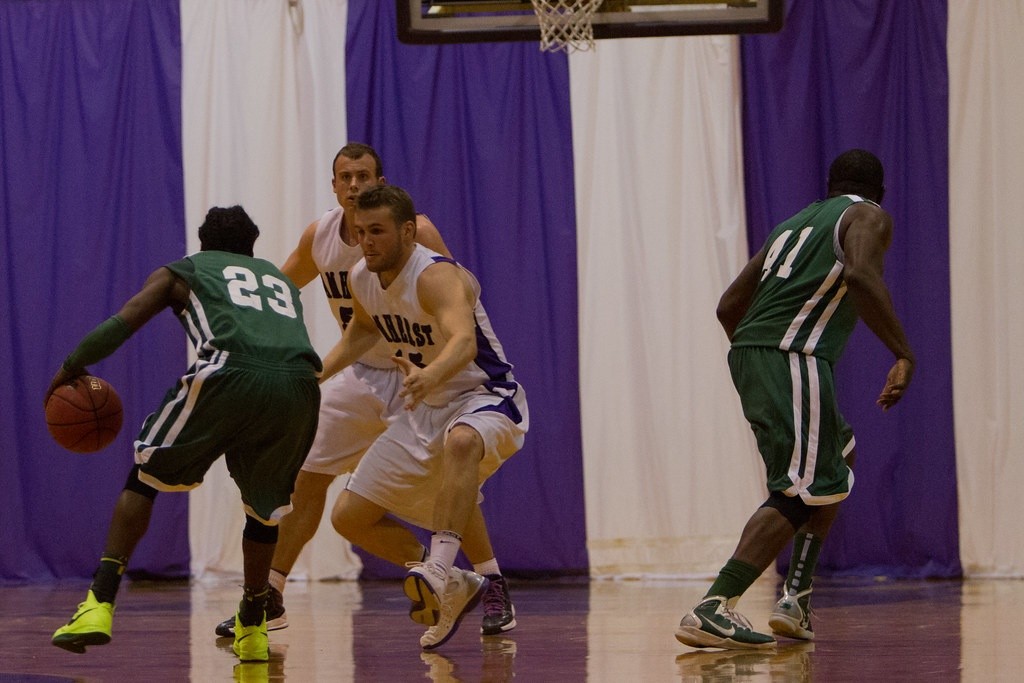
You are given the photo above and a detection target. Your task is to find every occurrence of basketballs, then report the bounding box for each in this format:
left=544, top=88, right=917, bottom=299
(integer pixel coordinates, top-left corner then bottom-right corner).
left=45, top=375, right=125, bottom=454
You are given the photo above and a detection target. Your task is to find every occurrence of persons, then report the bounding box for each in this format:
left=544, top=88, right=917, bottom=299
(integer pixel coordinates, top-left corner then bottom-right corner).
left=215, top=143, right=517, bottom=636
left=45, top=205, right=324, bottom=661
left=315, top=183, right=530, bottom=650
left=674, top=149, right=918, bottom=648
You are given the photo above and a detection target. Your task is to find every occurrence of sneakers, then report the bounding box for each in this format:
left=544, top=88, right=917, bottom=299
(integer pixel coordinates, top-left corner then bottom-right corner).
left=769, top=580, right=816, bottom=642
left=420, top=569, right=489, bottom=649
left=215, top=595, right=288, bottom=636
left=674, top=595, right=776, bottom=649
left=404, top=562, right=446, bottom=626
left=771, top=653, right=810, bottom=683
left=233, top=610, right=270, bottom=661
left=52, top=589, right=116, bottom=651
left=481, top=637, right=518, bottom=683
left=421, top=651, right=460, bottom=683
left=478, top=573, right=518, bottom=634
left=675, top=650, right=777, bottom=679
